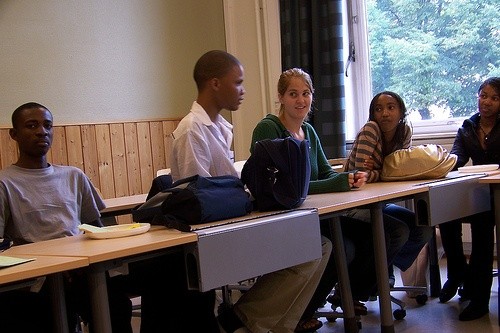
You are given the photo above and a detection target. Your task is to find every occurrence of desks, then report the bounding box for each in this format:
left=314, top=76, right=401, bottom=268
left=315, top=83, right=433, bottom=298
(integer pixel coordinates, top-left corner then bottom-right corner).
left=0, top=167, right=500, bottom=333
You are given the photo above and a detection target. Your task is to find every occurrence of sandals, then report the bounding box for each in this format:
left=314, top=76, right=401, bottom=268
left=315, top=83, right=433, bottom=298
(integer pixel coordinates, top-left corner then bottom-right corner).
left=328, top=292, right=368, bottom=315
left=295, top=317, right=322, bottom=333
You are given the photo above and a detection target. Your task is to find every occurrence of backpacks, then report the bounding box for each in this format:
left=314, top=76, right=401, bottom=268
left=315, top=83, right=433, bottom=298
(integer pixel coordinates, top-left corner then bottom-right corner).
left=241, top=138, right=311, bottom=209
left=131, top=174, right=250, bottom=226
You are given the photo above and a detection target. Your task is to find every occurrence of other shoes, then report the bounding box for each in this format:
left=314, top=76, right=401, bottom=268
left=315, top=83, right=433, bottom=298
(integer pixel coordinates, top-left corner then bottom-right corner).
left=460, top=301, right=489, bottom=321
left=439, top=280, right=458, bottom=302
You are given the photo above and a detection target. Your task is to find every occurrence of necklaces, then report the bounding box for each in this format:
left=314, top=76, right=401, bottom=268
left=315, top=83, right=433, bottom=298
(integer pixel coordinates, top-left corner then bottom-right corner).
left=480, top=126, right=488, bottom=144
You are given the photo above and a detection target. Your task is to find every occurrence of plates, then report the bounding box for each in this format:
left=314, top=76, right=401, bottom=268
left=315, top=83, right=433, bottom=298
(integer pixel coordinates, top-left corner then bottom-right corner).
left=457, top=164, right=499, bottom=173
left=86, top=223, right=151, bottom=239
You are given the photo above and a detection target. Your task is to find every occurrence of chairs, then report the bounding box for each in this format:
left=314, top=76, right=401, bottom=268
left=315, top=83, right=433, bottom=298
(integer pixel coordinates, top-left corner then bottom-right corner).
left=328, top=159, right=428, bottom=320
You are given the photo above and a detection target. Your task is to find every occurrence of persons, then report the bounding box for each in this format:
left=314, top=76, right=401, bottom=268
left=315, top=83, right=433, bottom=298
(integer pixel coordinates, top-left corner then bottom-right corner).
left=438, top=76, right=500, bottom=321
left=0, top=102, right=132, bottom=333
left=251, top=68, right=373, bottom=333
left=341, top=90, right=432, bottom=315
left=171, top=51, right=332, bottom=333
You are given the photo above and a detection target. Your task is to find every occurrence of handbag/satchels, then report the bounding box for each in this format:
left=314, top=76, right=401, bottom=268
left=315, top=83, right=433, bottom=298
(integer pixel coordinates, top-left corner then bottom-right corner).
left=379, top=143, right=458, bottom=182
